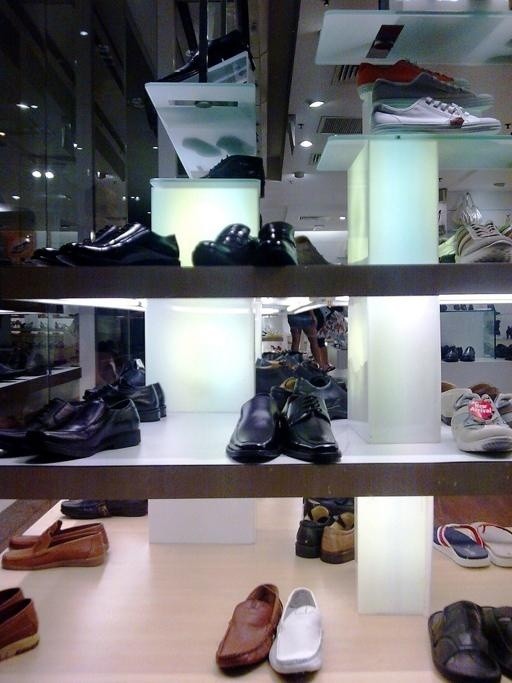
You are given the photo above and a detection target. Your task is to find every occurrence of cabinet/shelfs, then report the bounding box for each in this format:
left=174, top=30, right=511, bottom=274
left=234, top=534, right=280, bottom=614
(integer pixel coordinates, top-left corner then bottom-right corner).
left=314, top=133, right=512, bottom=619
left=145, top=1, right=257, bottom=178
left=145, top=177, right=262, bottom=546
left=1, top=302, right=143, bottom=552
left=313, top=0, right=512, bottom=73
left=1, top=263, right=512, bottom=683
left=441, top=307, right=508, bottom=402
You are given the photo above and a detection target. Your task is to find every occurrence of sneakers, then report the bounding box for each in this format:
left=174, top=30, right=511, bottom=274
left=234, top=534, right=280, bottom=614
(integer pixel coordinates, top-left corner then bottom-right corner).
left=438, top=219, right=512, bottom=262
left=441, top=381, right=473, bottom=425
left=453, top=220, right=512, bottom=264
left=470, top=383, right=511, bottom=428
left=356, top=60, right=471, bottom=101
left=199, top=154, right=265, bottom=197
left=370, top=71, right=496, bottom=113
left=451, top=393, right=512, bottom=453
left=367, top=96, right=501, bottom=135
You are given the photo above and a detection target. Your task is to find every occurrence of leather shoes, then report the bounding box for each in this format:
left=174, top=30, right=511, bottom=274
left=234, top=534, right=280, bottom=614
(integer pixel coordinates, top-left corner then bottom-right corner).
left=47, top=221, right=180, bottom=267
left=496, top=343, right=511, bottom=362
left=24, top=364, right=47, bottom=376
left=8, top=520, right=109, bottom=553
left=0, top=398, right=91, bottom=454
left=277, top=391, right=342, bottom=466
left=66, top=380, right=166, bottom=423
left=0, top=587, right=23, bottom=613
left=1, top=529, right=105, bottom=570
left=460, top=346, right=475, bottom=363
left=60, top=498, right=148, bottom=519
left=268, top=589, right=324, bottom=674
left=224, top=393, right=278, bottom=461
left=441, top=345, right=462, bottom=362
left=255, top=351, right=347, bottom=419
left=244, top=221, right=298, bottom=266
left=319, top=512, right=355, bottom=564
left=0, top=598, right=39, bottom=662
left=0, top=363, right=27, bottom=380
left=214, top=584, right=280, bottom=669
left=155, top=29, right=254, bottom=81
left=27, top=398, right=140, bottom=462
left=295, top=505, right=330, bottom=559
left=83, top=358, right=145, bottom=399
left=192, top=225, right=260, bottom=267
left=31, top=222, right=134, bottom=266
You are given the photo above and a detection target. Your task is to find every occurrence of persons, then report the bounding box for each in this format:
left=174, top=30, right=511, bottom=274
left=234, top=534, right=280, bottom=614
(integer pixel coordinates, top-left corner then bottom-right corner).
left=286, top=306, right=348, bottom=373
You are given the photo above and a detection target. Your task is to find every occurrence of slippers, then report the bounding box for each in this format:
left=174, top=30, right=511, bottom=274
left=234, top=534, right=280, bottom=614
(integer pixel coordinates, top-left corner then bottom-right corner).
left=433, top=523, right=490, bottom=568
left=469, top=521, right=512, bottom=568
left=427, top=600, right=502, bottom=683
left=482, top=605, right=512, bottom=681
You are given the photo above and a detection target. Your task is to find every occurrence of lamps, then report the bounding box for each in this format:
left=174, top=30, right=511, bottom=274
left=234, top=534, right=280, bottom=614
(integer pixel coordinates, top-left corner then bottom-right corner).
left=300, top=138, right=313, bottom=148
left=308, top=97, right=323, bottom=110
left=295, top=171, right=304, bottom=186
